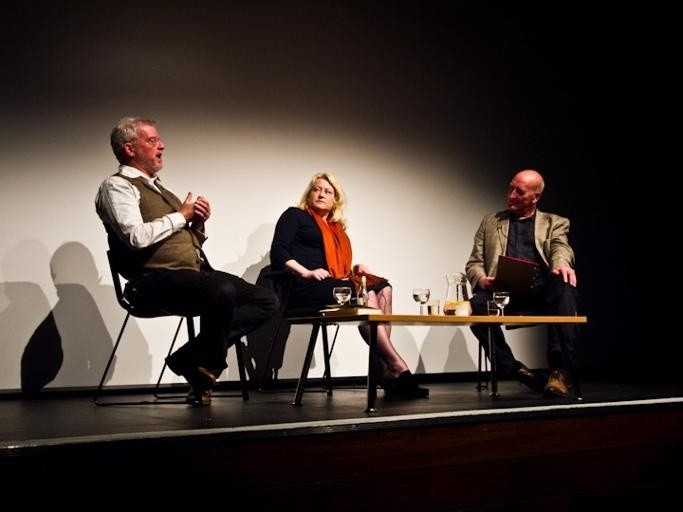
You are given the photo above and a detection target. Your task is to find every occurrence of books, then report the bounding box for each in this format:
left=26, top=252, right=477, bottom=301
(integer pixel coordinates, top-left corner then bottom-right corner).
left=492, top=253, right=538, bottom=296
left=318, top=303, right=377, bottom=316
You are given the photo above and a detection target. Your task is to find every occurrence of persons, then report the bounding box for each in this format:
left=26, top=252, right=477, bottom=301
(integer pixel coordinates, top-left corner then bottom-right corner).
left=95, top=116, right=280, bottom=406
left=271, top=172, right=429, bottom=399
left=466, top=171, right=585, bottom=403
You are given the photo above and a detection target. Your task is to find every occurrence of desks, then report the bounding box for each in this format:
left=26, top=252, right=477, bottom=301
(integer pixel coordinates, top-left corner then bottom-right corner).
left=278, top=307, right=591, bottom=400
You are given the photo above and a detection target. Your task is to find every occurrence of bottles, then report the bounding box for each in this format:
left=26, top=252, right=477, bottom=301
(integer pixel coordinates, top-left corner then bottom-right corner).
left=356, top=275, right=369, bottom=306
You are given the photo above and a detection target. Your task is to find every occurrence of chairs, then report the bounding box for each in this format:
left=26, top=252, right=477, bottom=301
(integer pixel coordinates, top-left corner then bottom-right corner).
left=460, top=271, right=577, bottom=399
left=91, top=246, right=255, bottom=405
left=260, top=265, right=361, bottom=411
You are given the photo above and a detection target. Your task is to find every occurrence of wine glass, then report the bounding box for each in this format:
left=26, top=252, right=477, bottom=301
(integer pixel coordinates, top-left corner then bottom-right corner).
left=492, top=290, right=511, bottom=317
left=411, top=287, right=430, bottom=316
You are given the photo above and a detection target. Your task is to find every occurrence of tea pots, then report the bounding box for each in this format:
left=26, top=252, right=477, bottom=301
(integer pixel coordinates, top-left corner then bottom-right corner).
left=442, top=271, right=475, bottom=316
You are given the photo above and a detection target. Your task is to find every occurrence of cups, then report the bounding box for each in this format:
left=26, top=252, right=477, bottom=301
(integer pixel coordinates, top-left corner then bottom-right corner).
left=332, top=286, right=351, bottom=305
left=428, top=299, right=439, bottom=315
left=484, top=299, right=500, bottom=316
